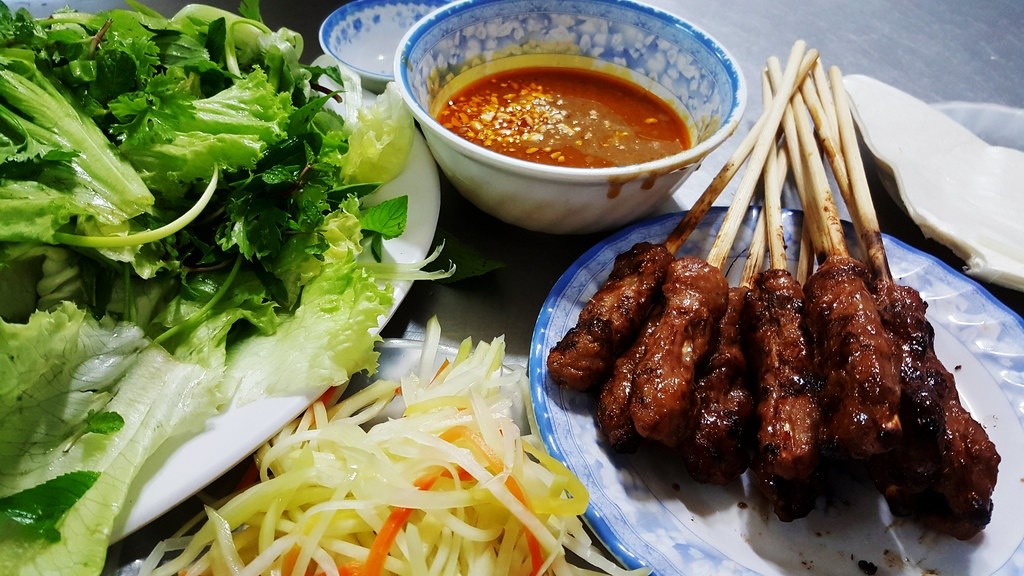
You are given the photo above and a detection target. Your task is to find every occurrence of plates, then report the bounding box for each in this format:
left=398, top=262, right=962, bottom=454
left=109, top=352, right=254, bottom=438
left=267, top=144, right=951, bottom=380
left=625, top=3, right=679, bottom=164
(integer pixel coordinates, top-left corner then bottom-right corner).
left=360, top=341, right=527, bottom=438
left=0, top=71, right=440, bottom=541
left=529, top=207, right=1024, bottom=576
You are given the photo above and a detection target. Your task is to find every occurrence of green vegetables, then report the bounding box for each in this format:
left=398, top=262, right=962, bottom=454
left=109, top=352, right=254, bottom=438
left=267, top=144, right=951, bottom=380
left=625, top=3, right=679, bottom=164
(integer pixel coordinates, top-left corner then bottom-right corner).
left=0, top=2, right=415, bottom=576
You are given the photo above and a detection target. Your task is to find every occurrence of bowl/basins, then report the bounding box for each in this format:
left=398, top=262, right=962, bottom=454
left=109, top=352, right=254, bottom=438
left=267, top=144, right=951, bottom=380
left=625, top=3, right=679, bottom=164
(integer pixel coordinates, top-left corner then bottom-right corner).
left=879, top=103, right=1024, bottom=210
left=318, top=0, right=460, bottom=95
left=396, top=0, right=748, bottom=235
